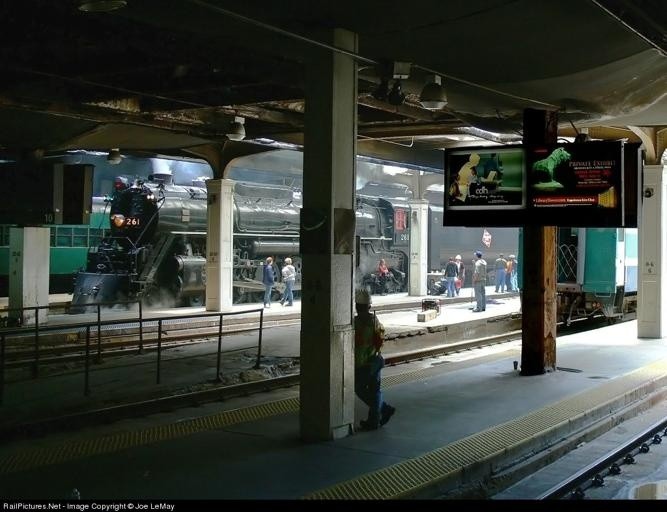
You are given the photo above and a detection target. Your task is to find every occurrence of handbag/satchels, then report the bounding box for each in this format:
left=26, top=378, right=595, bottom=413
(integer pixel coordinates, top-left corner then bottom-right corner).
left=276, top=283, right=286, bottom=293
left=455, top=280, right=461, bottom=288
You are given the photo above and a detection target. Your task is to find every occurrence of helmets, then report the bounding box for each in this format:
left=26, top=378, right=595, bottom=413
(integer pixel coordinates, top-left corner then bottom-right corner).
left=356, top=289, right=372, bottom=304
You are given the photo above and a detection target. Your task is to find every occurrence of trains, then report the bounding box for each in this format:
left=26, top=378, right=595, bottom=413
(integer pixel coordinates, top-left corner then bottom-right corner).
left=68, top=171, right=523, bottom=314
left=0, top=196, right=113, bottom=276
left=515, top=226, right=637, bottom=330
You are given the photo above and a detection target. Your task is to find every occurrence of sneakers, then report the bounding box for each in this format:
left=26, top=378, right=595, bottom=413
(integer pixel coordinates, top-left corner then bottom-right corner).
left=473, top=308, right=482, bottom=312
left=280, top=301, right=283, bottom=305
left=359, top=418, right=381, bottom=428
left=380, top=402, right=395, bottom=425
left=495, top=289, right=518, bottom=293
left=482, top=309, right=485, bottom=311
left=285, top=304, right=292, bottom=306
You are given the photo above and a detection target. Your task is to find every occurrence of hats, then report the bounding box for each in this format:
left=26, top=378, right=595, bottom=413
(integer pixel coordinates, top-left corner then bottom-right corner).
left=455, top=255, right=461, bottom=259
left=474, top=252, right=482, bottom=257
left=285, top=258, right=292, bottom=265
left=500, top=254, right=515, bottom=258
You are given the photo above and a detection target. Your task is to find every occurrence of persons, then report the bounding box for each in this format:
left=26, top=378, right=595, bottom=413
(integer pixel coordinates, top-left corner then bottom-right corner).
left=442, top=249, right=519, bottom=314
left=465, top=165, right=480, bottom=198
left=260, top=255, right=277, bottom=309
left=448, top=172, right=464, bottom=204
left=377, top=257, right=389, bottom=296
left=457, top=153, right=486, bottom=196
left=278, top=256, right=296, bottom=308
left=354, top=289, right=396, bottom=431
